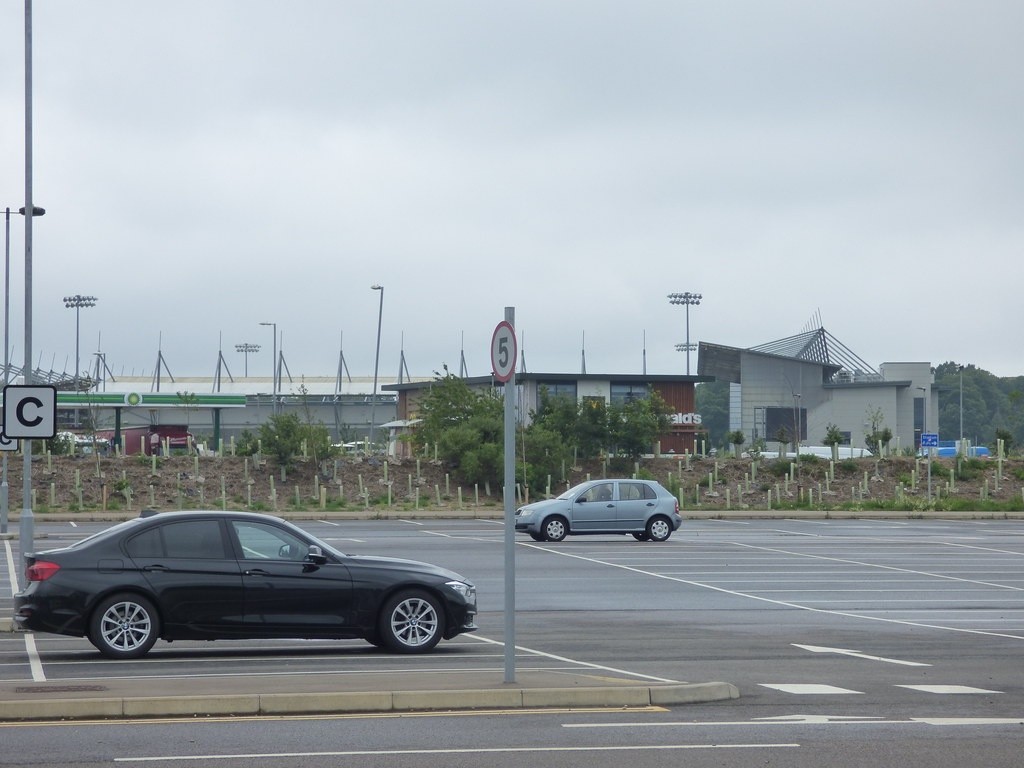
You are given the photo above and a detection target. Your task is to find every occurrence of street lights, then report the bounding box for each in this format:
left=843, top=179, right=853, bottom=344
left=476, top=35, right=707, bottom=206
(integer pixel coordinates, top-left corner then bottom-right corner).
left=958, top=364, right=965, bottom=452
left=793, top=393, right=803, bottom=449
left=667, top=292, right=703, bottom=375
left=235, top=343, right=261, bottom=379
left=61, top=293, right=96, bottom=396
left=369, top=284, right=384, bottom=457
left=915, top=386, right=927, bottom=435
left=258, top=322, right=278, bottom=420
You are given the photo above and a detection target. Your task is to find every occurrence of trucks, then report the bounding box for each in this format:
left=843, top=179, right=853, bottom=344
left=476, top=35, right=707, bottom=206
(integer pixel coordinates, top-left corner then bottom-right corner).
left=89, top=423, right=200, bottom=458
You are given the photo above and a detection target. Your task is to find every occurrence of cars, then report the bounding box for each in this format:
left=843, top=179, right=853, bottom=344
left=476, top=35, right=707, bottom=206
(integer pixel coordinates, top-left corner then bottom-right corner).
left=513, top=478, right=683, bottom=545
left=12, top=509, right=480, bottom=662
left=326, top=441, right=386, bottom=455
left=51, top=431, right=110, bottom=454
